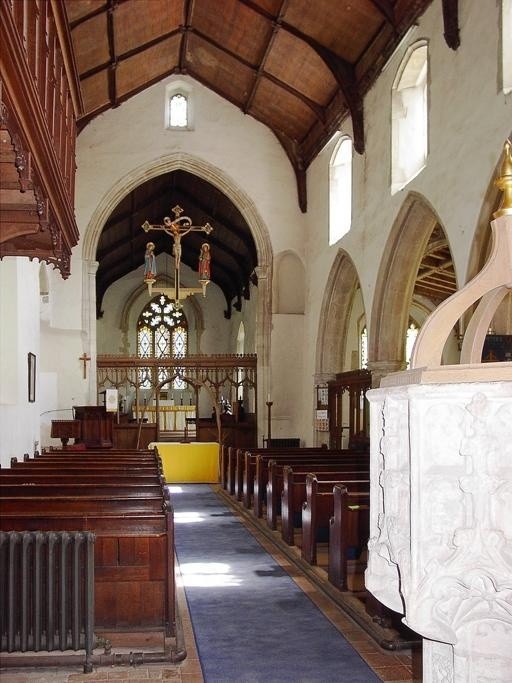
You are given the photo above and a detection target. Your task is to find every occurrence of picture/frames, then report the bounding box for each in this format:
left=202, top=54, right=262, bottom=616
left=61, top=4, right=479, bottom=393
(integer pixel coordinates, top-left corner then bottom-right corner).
left=28, top=352, right=36, bottom=402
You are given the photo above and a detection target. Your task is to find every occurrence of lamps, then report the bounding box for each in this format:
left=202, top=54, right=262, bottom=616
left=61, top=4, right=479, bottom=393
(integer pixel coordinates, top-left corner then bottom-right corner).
left=141, top=205, right=214, bottom=310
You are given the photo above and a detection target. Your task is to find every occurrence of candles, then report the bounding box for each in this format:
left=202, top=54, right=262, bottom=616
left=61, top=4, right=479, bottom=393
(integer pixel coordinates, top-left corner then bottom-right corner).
left=144, top=392, right=146, bottom=399
left=154, top=394, right=156, bottom=399
left=268, top=394, right=271, bottom=402
left=180, top=393, right=182, bottom=399
left=124, top=395, right=126, bottom=399
left=134, top=393, right=136, bottom=398
left=171, top=393, right=173, bottom=399
left=190, top=393, right=192, bottom=398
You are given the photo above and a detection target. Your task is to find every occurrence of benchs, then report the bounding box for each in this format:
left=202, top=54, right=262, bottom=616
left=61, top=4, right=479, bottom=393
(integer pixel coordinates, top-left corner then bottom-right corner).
left=221, top=444, right=370, bottom=591
left=0, top=511, right=175, bottom=645
left=0, top=446, right=172, bottom=509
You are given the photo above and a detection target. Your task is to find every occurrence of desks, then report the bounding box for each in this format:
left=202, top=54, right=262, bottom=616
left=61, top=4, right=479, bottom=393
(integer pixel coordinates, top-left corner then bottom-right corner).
left=132, top=406, right=197, bottom=438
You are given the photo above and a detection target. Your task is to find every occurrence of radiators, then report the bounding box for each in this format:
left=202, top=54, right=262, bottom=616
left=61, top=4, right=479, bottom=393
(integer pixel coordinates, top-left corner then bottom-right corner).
left=0, top=531, right=95, bottom=672
